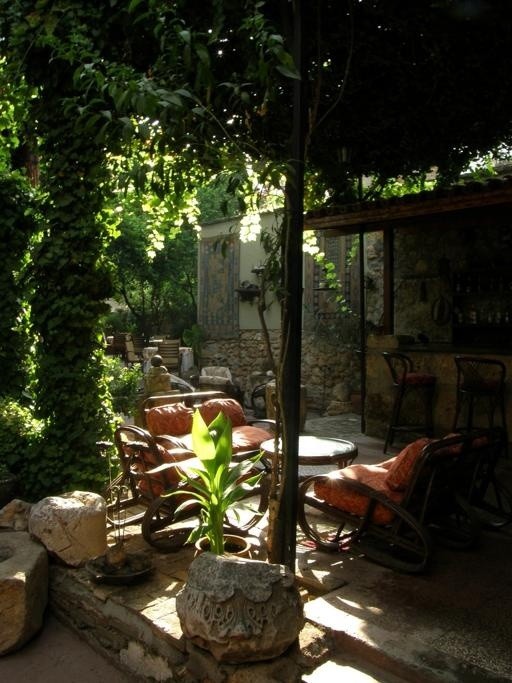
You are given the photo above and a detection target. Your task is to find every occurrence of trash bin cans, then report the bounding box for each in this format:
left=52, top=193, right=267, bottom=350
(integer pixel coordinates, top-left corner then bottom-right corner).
left=266, top=379, right=308, bottom=432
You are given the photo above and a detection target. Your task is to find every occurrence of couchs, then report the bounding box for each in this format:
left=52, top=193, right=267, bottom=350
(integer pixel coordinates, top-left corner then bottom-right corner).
left=101, top=391, right=283, bottom=554
left=296, top=426, right=511, bottom=576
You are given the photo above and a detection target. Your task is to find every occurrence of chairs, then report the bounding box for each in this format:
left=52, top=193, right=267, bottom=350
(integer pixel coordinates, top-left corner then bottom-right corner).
left=452, top=354, right=509, bottom=460
left=381, top=352, right=437, bottom=453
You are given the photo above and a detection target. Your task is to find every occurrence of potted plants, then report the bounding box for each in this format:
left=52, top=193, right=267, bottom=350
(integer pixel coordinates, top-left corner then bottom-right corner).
left=143, top=404, right=267, bottom=558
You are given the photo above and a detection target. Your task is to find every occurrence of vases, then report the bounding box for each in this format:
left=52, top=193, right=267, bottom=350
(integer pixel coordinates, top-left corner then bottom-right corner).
left=86, top=552, right=154, bottom=585
left=351, top=392, right=361, bottom=405
